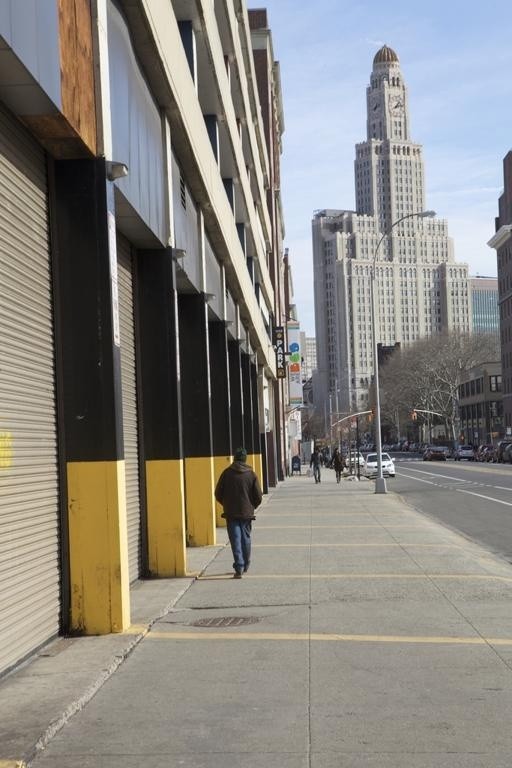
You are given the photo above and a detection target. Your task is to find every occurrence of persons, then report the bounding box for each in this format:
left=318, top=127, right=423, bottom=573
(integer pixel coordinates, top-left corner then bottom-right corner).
left=310, top=446, right=324, bottom=483
left=214, top=446, right=262, bottom=577
left=328, top=447, right=346, bottom=483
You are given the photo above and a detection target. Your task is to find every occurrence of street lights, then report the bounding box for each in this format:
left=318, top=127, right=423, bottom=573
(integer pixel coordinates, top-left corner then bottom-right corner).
left=367, top=209, right=438, bottom=494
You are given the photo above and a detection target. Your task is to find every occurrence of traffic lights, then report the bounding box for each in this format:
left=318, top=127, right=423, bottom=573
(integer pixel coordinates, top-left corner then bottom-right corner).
left=412, top=412, right=418, bottom=420
left=367, top=416, right=372, bottom=422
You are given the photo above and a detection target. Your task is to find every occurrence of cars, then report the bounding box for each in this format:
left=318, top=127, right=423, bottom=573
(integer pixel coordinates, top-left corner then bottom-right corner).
left=325, top=439, right=512, bottom=481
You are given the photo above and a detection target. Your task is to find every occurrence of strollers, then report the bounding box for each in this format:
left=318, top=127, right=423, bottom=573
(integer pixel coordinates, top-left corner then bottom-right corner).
left=290, top=455, right=301, bottom=476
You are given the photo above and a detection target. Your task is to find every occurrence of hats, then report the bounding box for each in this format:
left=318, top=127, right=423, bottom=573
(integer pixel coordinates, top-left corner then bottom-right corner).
left=234, top=448, right=246, bottom=461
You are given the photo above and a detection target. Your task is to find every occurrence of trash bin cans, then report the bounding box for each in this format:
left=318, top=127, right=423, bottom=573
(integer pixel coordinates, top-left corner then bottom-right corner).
left=291, top=455, right=301, bottom=475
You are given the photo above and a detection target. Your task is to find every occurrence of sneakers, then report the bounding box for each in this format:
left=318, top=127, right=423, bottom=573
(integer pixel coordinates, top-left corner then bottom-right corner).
left=244, top=567, right=248, bottom=572
left=234, top=571, right=242, bottom=577
